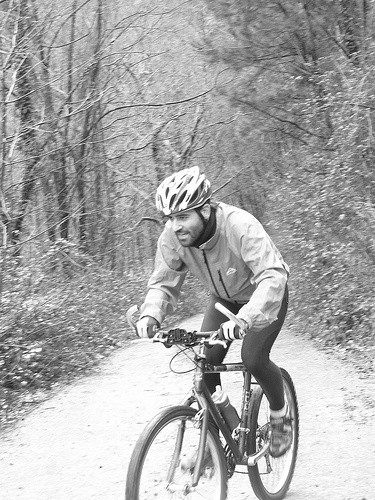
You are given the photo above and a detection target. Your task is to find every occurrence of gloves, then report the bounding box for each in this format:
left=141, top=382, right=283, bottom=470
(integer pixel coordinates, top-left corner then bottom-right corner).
left=220, top=319, right=249, bottom=341
left=135, top=317, right=160, bottom=338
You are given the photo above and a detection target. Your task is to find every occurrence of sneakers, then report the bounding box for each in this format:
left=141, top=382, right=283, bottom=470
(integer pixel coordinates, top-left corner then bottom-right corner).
left=269, top=403, right=293, bottom=458
left=182, top=449, right=213, bottom=472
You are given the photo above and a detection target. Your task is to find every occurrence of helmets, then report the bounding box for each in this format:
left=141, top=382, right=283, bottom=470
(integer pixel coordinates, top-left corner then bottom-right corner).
left=156, top=166, right=213, bottom=216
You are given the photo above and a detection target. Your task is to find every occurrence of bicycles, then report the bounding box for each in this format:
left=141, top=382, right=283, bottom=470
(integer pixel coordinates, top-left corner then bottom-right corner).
left=124, top=302, right=300, bottom=500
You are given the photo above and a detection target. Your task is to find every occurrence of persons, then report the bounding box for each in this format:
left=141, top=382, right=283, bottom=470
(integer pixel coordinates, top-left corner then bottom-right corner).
left=135, top=165, right=293, bottom=470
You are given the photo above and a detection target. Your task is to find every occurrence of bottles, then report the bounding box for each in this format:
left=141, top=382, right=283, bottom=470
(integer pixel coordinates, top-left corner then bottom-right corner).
left=212, top=385, right=240, bottom=430
